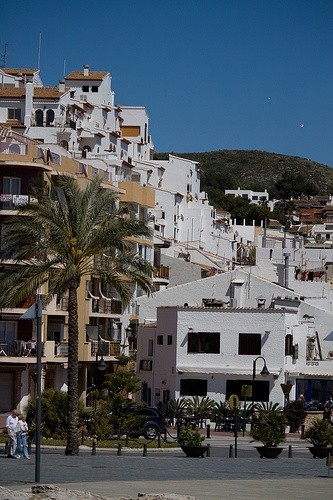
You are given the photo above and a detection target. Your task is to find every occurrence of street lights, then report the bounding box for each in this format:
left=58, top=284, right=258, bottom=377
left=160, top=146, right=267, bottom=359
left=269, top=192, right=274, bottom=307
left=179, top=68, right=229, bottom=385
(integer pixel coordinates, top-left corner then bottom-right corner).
left=250, top=356, right=271, bottom=436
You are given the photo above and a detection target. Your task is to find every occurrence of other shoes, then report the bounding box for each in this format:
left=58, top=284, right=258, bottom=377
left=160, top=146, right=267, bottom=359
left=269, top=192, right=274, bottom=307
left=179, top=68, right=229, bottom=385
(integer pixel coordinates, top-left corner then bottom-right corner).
left=17, top=455, right=22, bottom=457
left=7, top=455, right=17, bottom=459
left=25, top=456, right=30, bottom=459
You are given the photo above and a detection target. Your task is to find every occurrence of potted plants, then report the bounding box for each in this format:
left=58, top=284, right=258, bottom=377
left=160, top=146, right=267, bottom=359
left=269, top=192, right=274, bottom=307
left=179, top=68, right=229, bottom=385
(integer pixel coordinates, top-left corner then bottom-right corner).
left=248, top=412, right=288, bottom=458
left=299, top=418, right=333, bottom=459
left=177, top=425, right=208, bottom=458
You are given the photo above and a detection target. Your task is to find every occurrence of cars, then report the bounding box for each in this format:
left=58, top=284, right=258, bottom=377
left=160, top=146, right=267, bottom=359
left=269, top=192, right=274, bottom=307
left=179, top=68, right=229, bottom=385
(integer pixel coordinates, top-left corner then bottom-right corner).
left=83, top=403, right=166, bottom=441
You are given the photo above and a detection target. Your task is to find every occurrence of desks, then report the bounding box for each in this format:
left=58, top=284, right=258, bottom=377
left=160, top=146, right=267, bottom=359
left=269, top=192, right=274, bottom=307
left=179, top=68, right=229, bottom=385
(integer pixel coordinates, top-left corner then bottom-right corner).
left=0, top=343, right=7, bottom=357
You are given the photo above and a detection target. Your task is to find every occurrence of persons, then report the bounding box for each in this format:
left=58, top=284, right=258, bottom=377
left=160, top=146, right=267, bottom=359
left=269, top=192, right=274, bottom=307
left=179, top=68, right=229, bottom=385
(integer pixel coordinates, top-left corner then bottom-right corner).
left=296, top=393, right=333, bottom=421
left=6, top=409, right=31, bottom=459
